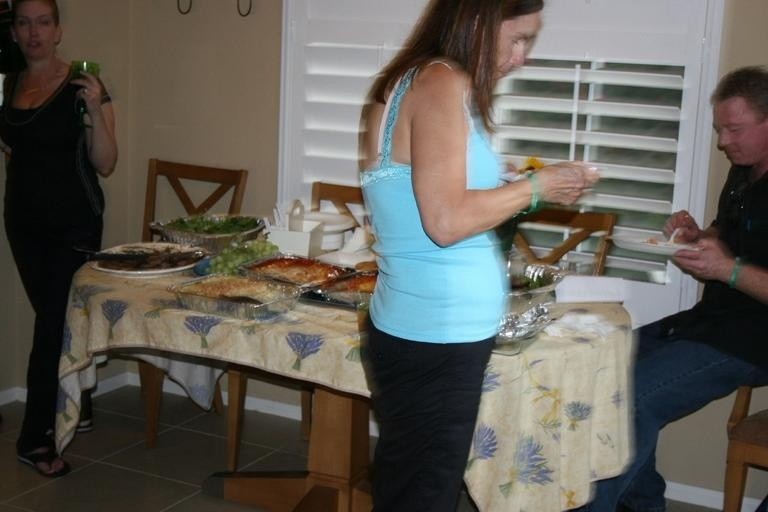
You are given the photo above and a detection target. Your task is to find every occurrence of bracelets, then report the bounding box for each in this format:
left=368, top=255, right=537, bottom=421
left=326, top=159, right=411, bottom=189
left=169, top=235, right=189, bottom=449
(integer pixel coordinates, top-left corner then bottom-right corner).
left=727, top=255, right=742, bottom=289
left=520, top=172, right=543, bottom=215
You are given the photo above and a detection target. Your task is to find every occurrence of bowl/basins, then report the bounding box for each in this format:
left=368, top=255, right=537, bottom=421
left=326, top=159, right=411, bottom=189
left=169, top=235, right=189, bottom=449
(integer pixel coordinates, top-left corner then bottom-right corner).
left=646, top=268, right=665, bottom=282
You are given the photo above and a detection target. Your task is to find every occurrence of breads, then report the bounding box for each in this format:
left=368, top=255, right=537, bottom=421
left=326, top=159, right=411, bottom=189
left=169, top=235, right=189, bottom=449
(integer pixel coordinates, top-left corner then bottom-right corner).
left=175, top=251, right=376, bottom=315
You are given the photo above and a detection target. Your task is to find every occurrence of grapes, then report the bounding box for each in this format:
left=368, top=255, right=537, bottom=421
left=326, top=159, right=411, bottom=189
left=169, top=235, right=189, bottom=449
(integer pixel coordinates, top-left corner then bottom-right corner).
left=203, top=240, right=280, bottom=275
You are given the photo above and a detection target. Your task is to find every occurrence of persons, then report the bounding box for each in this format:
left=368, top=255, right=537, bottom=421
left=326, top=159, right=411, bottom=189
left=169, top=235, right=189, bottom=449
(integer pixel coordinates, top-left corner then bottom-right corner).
left=358, top=0, right=600, bottom=511
left=0, top=1, right=119, bottom=479
left=566, top=66, right=767, bottom=512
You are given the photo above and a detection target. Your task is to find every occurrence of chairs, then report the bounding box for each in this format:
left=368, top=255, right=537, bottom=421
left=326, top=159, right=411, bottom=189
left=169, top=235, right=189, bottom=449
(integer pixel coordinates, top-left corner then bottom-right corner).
left=140, top=158, right=249, bottom=471
left=302, top=182, right=369, bottom=445
left=728, top=387, right=768, bottom=512
left=510, top=209, right=618, bottom=289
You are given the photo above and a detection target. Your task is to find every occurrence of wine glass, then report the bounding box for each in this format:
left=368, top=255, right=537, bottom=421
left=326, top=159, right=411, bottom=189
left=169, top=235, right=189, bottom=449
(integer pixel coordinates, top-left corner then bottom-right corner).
left=69, top=59, right=101, bottom=129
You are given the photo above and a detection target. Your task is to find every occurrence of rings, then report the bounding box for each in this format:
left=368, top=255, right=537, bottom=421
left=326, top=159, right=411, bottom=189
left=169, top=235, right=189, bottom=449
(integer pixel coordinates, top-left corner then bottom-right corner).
left=81, top=88, right=88, bottom=94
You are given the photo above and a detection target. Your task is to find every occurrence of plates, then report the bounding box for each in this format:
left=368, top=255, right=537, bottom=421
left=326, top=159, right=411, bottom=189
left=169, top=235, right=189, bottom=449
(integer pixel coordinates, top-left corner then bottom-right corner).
left=500, top=164, right=601, bottom=182
left=90, top=241, right=203, bottom=279
left=292, top=209, right=355, bottom=251
left=605, top=232, right=699, bottom=260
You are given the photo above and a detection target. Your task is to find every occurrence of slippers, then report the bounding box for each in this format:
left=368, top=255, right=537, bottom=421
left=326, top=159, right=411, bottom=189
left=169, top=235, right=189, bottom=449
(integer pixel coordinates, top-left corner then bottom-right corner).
left=17, top=447, right=71, bottom=478
